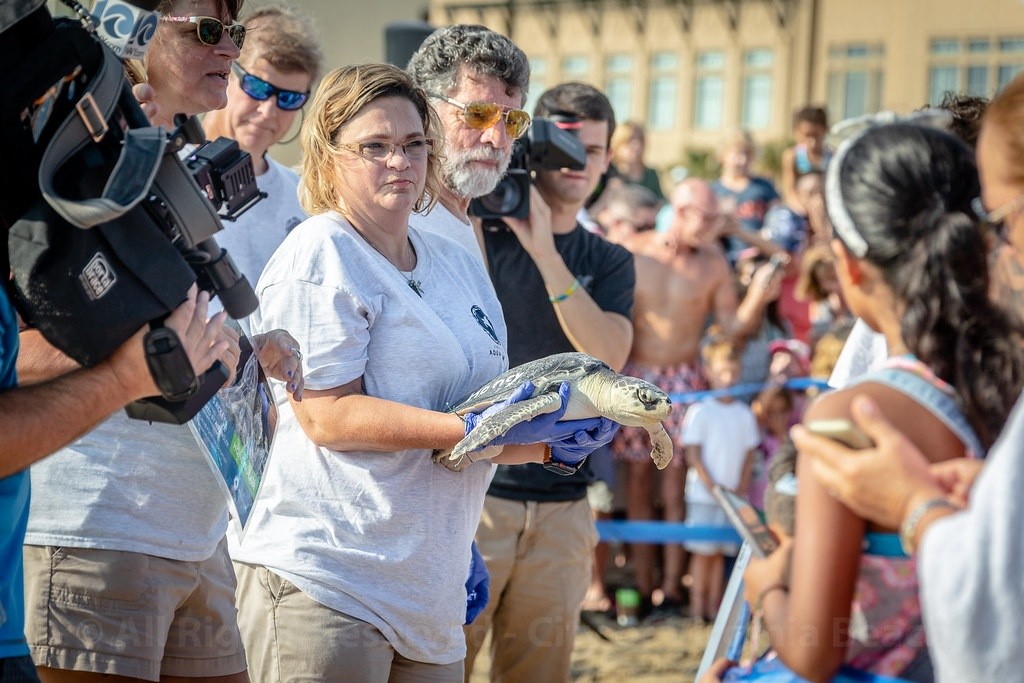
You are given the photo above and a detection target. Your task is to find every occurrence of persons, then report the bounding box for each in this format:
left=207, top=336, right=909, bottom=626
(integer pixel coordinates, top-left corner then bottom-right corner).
left=581, top=102, right=837, bottom=618
left=231, top=64, right=620, bottom=683
left=175, top=6, right=310, bottom=319
left=790, top=72, right=1024, bottom=683
left=743, top=108, right=1024, bottom=683
left=408, top=24, right=530, bottom=257
left=0, top=0, right=305, bottom=683
left=463, top=82, right=634, bottom=683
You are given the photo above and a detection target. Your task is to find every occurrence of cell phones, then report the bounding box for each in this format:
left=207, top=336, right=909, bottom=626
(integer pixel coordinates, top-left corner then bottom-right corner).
left=712, top=483, right=782, bottom=559
left=807, top=421, right=875, bottom=449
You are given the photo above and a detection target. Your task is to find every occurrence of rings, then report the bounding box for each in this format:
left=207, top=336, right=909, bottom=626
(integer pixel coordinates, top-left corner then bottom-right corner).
left=294, top=348, right=301, bottom=360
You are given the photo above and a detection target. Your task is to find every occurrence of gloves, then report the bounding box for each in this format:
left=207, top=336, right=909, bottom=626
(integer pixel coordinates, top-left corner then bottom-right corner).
left=465, top=380, right=601, bottom=453
left=546, top=417, right=621, bottom=463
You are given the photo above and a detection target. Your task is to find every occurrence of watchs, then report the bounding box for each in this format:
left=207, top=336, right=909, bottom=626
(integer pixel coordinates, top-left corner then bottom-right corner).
left=543, top=444, right=586, bottom=476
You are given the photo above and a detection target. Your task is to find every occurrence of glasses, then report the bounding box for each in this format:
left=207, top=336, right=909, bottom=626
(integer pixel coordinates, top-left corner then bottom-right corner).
left=230, top=60, right=311, bottom=110
left=974, top=195, right=1024, bottom=245
left=158, top=15, right=246, bottom=50
left=329, top=139, right=425, bottom=160
left=426, top=93, right=531, bottom=139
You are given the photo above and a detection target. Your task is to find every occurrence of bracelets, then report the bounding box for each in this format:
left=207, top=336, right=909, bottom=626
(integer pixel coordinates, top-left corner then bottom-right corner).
left=898, top=499, right=946, bottom=554
left=755, top=583, right=787, bottom=610
left=549, top=279, right=579, bottom=304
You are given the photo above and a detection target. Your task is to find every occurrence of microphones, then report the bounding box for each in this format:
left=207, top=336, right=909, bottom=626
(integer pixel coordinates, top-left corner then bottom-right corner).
left=202, top=249, right=260, bottom=321
